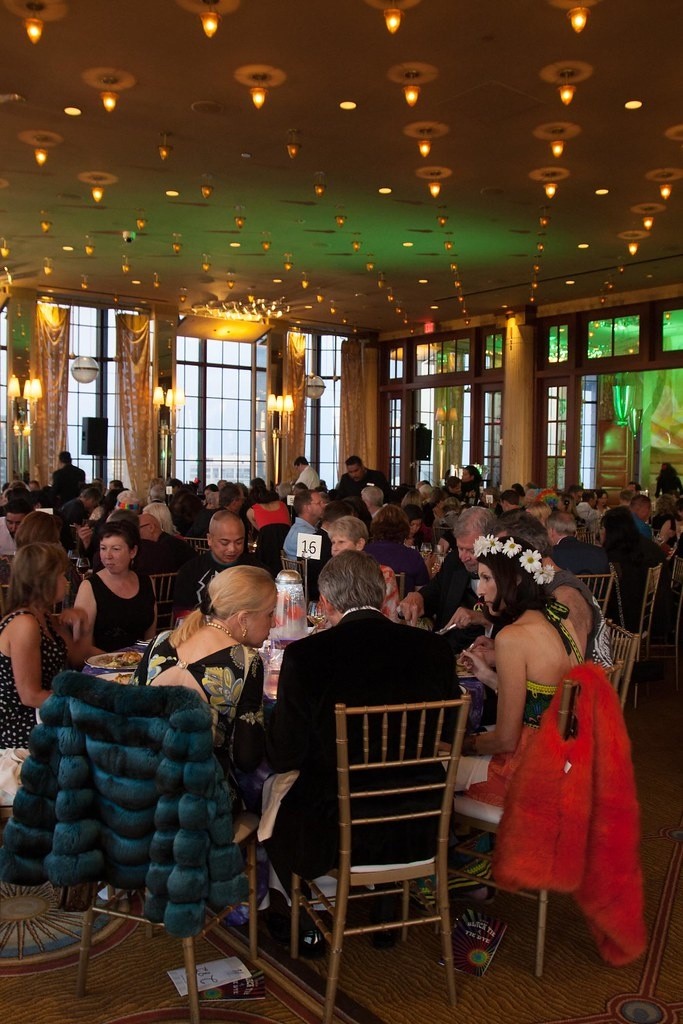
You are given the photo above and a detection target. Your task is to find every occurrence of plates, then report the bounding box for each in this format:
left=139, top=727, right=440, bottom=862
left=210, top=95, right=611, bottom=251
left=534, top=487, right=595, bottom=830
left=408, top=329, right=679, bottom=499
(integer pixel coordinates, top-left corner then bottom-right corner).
left=96, top=672, right=134, bottom=685
left=84, top=652, right=144, bottom=669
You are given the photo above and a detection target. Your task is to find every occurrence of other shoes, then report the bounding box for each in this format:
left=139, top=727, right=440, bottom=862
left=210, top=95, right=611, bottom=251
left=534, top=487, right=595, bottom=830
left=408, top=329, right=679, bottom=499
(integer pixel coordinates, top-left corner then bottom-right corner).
left=266, top=888, right=292, bottom=938
left=463, top=889, right=496, bottom=904
left=368, top=916, right=395, bottom=948
left=410, top=896, right=437, bottom=916
left=301, top=933, right=324, bottom=958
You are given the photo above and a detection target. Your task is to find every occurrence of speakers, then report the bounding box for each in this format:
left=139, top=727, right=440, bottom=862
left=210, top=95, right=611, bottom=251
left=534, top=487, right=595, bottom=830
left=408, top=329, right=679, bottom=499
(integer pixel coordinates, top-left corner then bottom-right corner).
left=413, top=428, right=432, bottom=461
left=81, top=417, right=108, bottom=456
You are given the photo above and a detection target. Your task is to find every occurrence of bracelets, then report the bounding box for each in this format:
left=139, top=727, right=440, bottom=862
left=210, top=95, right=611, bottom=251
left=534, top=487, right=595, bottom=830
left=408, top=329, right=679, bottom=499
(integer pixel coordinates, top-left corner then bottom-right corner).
left=495, top=688, right=498, bottom=696
left=463, top=735, right=479, bottom=756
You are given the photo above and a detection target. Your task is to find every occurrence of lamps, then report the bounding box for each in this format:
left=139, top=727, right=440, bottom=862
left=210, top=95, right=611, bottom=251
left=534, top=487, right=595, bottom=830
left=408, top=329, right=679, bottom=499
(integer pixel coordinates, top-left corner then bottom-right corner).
left=447, top=409, right=458, bottom=440
left=275, top=396, right=285, bottom=431
left=23, top=374, right=43, bottom=437
left=152, top=387, right=185, bottom=435
left=71, top=354, right=100, bottom=382
left=267, top=393, right=294, bottom=439
left=7, top=374, right=24, bottom=436
left=191, top=294, right=283, bottom=324
left=434, top=407, right=447, bottom=445
left=164, top=389, right=174, bottom=432
left=304, top=372, right=326, bottom=398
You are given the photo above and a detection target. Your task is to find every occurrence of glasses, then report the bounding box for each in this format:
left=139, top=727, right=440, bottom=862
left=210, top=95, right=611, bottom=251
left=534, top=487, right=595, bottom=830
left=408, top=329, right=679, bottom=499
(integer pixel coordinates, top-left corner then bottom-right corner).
left=310, top=501, right=323, bottom=507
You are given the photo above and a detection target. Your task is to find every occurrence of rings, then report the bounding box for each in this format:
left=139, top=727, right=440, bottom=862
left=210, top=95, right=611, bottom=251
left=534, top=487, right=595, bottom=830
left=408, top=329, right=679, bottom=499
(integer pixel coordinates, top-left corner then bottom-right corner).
left=69, top=618, right=72, bottom=623
left=460, top=619, right=464, bottom=623
left=471, top=662, right=473, bottom=665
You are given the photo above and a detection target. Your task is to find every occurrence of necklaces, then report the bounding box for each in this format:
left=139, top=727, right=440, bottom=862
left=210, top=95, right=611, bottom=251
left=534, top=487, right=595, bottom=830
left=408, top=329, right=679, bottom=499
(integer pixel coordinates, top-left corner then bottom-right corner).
left=206, top=622, right=232, bottom=637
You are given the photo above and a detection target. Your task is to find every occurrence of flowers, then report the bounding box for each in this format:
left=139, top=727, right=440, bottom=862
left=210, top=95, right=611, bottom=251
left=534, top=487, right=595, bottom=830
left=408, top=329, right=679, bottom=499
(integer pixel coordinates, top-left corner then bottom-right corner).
left=471, top=535, right=556, bottom=585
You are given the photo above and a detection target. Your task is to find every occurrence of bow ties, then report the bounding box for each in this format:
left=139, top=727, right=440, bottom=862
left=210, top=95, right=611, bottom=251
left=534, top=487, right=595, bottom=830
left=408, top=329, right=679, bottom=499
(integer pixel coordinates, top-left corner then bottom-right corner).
left=471, top=572, right=479, bottom=579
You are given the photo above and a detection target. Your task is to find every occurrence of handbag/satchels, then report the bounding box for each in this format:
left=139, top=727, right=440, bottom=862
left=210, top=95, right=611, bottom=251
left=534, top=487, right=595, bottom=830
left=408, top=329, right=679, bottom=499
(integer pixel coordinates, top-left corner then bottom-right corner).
left=0, top=748, right=31, bottom=806
left=53, top=882, right=93, bottom=911
left=632, top=661, right=664, bottom=681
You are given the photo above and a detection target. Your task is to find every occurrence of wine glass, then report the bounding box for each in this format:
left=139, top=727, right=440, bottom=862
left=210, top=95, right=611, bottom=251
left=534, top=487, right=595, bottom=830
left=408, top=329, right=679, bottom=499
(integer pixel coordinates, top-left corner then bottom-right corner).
left=257, top=633, right=283, bottom=685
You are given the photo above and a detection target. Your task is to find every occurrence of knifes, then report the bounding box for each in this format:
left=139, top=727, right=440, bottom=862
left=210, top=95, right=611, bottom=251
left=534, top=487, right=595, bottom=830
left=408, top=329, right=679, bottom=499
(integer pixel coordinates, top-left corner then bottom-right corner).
left=435, top=624, right=456, bottom=635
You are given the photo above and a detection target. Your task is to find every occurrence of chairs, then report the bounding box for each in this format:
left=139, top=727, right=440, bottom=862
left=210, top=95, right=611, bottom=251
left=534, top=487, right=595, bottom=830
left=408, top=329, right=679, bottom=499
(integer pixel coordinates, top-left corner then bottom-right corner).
left=0, top=531, right=681, bottom=1024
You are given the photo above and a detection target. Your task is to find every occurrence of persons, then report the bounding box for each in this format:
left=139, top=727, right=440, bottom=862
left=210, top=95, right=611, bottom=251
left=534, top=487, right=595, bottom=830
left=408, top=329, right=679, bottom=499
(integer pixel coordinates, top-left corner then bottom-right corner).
left=285, top=456, right=683, bottom=648
left=256, top=549, right=472, bottom=959
left=481, top=507, right=614, bottom=687
left=390, top=539, right=588, bottom=904
left=169, top=510, right=274, bottom=620
left=73, top=520, right=158, bottom=651
left=395, top=506, right=498, bottom=657
left=129, top=564, right=278, bottom=922
left=0, top=451, right=318, bottom=614
left=316, top=515, right=400, bottom=624
left=0, top=544, right=106, bottom=751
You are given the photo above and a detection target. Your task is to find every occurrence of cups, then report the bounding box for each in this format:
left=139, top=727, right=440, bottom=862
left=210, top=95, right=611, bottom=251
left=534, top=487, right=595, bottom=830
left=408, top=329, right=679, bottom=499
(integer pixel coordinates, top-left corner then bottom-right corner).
left=68, top=550, right=79, bottom=563
left=306, top=601, right=327, bottom=626
left=421, top=543, right=432, bottom=555
left=76, top=558, right=90, bottom=574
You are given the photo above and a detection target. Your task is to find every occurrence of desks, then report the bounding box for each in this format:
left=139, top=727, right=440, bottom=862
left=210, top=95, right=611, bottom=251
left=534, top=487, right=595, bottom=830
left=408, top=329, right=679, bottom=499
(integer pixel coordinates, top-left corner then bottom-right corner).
left=82, top=618, right=488, bottom=933
